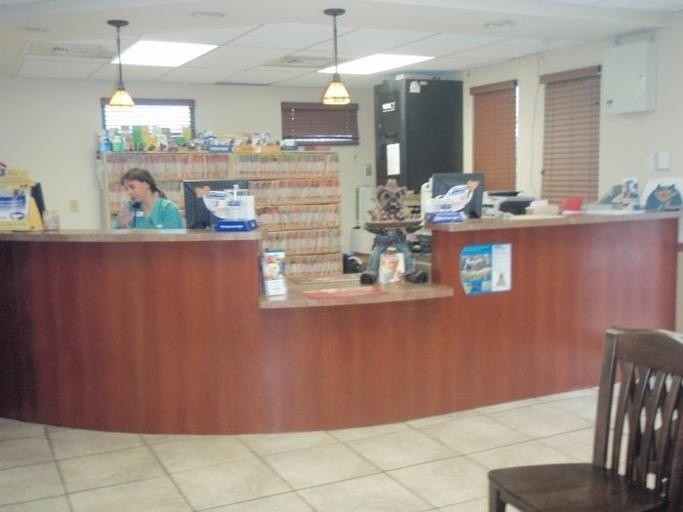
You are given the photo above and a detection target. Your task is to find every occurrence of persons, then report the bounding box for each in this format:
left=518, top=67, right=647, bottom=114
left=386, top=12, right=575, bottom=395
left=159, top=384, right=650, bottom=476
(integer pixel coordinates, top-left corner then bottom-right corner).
left=110, top=167, right=183, bottom=229
left=360, top=178, right=426, bottom=285
left=426, top=175, right=481, bottom=214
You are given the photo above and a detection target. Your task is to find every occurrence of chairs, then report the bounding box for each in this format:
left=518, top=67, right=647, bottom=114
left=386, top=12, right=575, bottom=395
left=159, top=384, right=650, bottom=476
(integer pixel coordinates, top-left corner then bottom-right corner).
left=486, top=327, right=683, bottom=512
left=624, top=333, right=683, bottom=512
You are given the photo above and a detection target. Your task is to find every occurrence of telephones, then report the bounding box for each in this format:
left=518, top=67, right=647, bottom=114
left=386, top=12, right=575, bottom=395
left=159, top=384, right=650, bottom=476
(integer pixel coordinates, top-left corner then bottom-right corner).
left=130, top=198, right=140, bottom=208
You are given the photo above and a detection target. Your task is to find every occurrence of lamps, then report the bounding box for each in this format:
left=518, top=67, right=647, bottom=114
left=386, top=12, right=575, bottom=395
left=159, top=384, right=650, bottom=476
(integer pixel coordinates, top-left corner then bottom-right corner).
left=108, top=18, right=134, bottom=107
left=324, top=6, right=351, bottom=104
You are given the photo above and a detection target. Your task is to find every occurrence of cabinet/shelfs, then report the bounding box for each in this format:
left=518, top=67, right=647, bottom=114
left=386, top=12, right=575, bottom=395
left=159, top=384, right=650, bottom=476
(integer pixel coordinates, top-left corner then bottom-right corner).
left=95, top=149, right=345, bottom=281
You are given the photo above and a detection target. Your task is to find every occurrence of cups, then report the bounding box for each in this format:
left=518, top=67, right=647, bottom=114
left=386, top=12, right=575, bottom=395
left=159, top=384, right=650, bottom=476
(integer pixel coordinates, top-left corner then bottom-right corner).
left=41, top=210, right=59, bottom=232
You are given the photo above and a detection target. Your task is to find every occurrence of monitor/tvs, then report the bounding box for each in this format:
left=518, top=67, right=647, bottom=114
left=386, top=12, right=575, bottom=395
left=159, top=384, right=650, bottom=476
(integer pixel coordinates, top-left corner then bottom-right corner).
left=183, top=180, right=249, bottom=228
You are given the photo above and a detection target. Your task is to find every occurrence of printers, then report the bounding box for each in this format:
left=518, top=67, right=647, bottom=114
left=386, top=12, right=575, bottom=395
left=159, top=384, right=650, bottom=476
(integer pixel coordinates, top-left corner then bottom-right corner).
left=482, top=190, right=536, bottom=215
